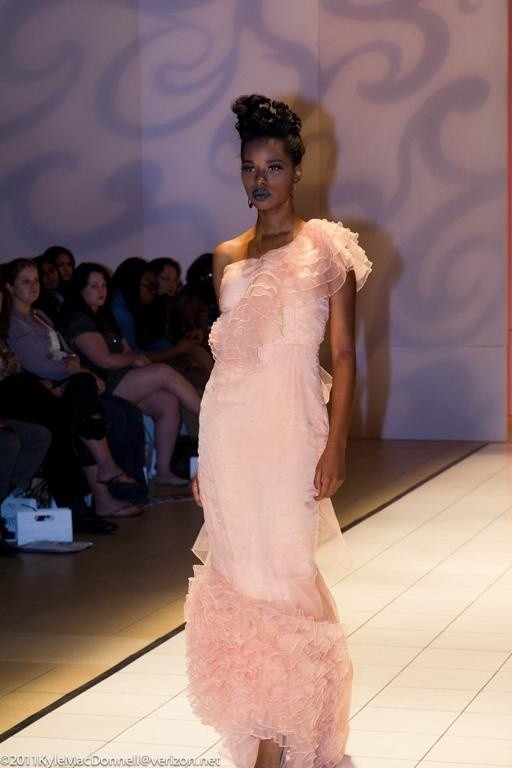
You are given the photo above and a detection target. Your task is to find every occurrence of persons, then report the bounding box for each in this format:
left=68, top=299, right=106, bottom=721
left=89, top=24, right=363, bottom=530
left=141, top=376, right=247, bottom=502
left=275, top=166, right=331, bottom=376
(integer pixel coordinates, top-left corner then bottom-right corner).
left=1, top=245, right=218, bottom=557
left=186, top=94, right=375, bottom=767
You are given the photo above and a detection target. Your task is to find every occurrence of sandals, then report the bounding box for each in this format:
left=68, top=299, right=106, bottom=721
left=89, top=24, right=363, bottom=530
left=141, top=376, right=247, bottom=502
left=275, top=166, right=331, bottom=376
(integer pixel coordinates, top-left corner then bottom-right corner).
left=157, top=475, right=189, bottom=486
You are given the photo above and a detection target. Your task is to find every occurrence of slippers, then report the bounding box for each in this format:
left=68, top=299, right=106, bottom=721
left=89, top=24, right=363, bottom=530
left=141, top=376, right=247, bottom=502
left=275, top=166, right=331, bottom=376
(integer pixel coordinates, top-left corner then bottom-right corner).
left=95, top=473, right=137, bottom=485
left=96, top=504, right=145, bottom=518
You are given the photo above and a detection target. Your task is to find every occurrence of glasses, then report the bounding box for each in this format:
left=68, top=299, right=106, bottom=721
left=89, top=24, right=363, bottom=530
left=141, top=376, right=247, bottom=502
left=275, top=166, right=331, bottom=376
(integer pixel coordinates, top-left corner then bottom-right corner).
left=143, top=282, right=160, bottom=292
